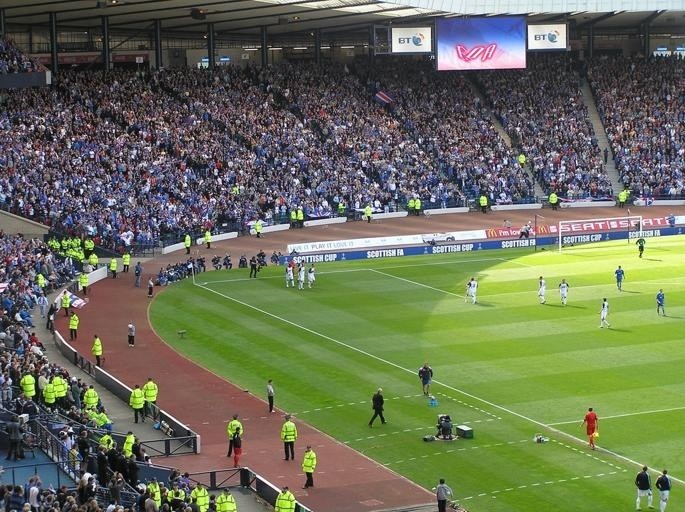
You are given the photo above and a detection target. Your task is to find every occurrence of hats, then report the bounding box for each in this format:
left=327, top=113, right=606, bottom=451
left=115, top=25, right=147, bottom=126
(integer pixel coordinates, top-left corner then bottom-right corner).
left=172, top=482, right=179, bottom=486
left=284, top=415, right=291, bottom=419
left=223, top=488, right=229, bottom=492
left=307, top=445, right=312, bottom=448
left=151, top=477, right=156, bottom=482
left=197, top=482, right=204, bottom=486
left=282, top=486, right=288, bottom=490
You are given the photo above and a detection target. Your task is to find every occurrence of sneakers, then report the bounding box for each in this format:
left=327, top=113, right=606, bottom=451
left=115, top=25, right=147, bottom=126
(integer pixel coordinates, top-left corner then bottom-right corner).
left=465, top=299, right=477, bottom=305
left=134, top=413, right=157, bottom=424
left=649, top=506, right=654, bottom=510
left=270, top=410, right=275, bottom=413
left=286, top=285, right=311, bottom=290
left=540, top=299, right=546, bottom=304
left=636, top=507, right=642, bottom=511
left=4, top=456, right=21, bottom=461
left=599, top=324, right=611, bottom=329
left=588, top=444, right=595, bottom=450
left=368, top=423, right=373, bottom=428
left=382, top=421, right=387, bottom=424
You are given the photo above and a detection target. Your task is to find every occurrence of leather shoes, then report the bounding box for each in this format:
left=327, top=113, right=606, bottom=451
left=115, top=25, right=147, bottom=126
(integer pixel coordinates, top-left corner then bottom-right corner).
left=282, top=457, right=289, bottom=460
left=291, top=456, right=295, bottom=460
left=302, top=484, right=314, bottom=489
